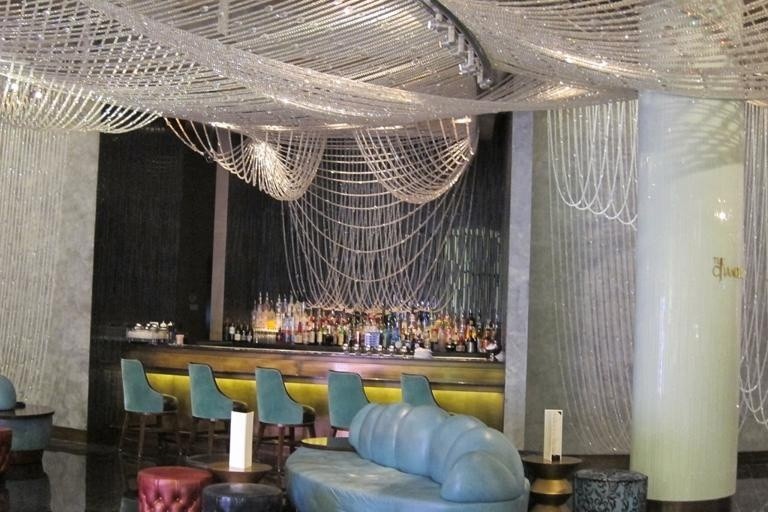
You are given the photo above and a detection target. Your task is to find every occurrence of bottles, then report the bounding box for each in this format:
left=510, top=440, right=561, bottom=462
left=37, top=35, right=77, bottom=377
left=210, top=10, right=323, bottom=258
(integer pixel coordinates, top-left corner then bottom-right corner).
left=224, top=292, right=497, bottom=354
left=132, top=320, right=174, bottom=344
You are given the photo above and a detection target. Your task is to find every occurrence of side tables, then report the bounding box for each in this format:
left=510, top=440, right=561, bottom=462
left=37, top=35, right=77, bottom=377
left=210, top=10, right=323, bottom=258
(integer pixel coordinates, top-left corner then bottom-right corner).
left=208, top=461, right=273, bottom=483
left=523, top=456, right=583, bottom=512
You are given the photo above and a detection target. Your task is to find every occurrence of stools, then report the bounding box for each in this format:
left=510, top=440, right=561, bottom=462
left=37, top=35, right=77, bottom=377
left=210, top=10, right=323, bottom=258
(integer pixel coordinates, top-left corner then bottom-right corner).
left=136, top=466, right=214, bottom=512
left=573, top=467, right=649, bottom=512
left=201, top=482, right=283, bottom=512
left=0, top=430, right=11, bottom=486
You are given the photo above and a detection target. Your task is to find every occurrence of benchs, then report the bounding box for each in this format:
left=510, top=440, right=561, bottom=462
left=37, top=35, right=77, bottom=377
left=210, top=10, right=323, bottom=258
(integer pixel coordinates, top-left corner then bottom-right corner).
left=284, top=402, right=531, bottom=512
